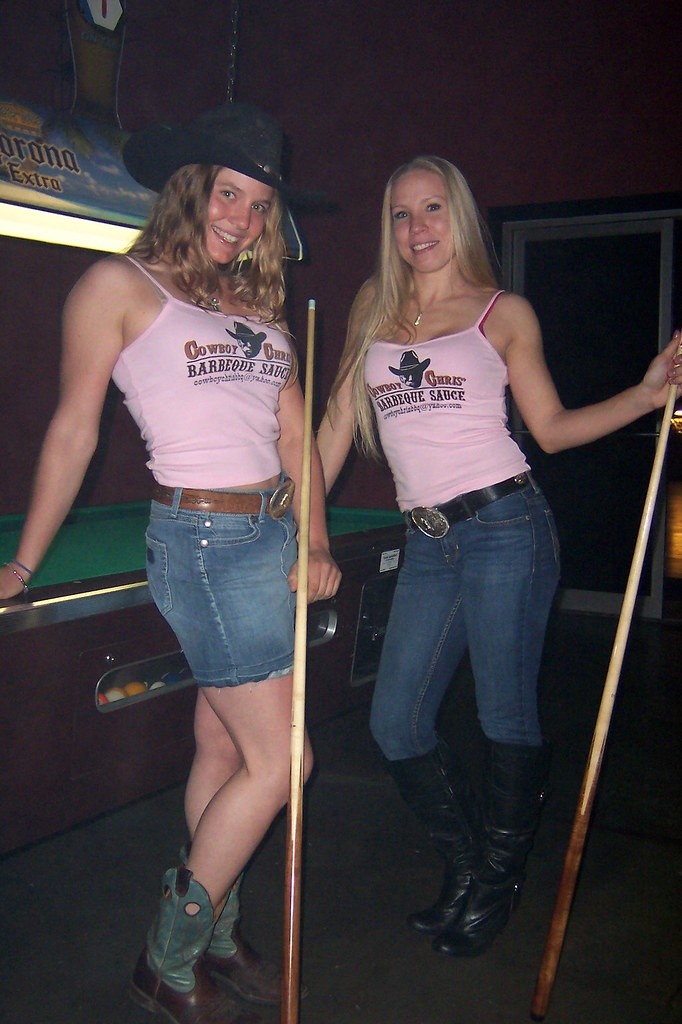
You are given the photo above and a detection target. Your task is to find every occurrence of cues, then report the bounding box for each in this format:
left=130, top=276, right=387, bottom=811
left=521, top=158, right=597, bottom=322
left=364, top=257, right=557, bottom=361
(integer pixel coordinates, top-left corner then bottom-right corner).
left=529, top=363, right=682, bottom=1023
left=280, top=292, right=317, bottom=1023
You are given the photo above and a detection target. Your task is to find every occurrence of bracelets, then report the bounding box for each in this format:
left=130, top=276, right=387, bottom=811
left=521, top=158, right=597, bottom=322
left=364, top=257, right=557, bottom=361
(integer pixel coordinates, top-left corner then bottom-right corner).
left=3, top=559, right=35, bottom=593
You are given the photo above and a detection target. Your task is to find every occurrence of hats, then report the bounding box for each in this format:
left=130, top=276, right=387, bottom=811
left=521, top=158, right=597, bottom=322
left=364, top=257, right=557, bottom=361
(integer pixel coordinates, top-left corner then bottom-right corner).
left=177, top=101, right=284, bottom=189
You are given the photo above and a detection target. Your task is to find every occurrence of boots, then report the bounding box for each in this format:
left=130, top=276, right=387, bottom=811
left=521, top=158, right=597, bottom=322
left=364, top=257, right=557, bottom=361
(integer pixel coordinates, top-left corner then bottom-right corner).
left=399, top=759, right=488, bottom=936
left=179, top=842, right=309, bottom=1007
left=431, top=741, right=570, bottom=958
left=127, top=863, right=264, bottom=1024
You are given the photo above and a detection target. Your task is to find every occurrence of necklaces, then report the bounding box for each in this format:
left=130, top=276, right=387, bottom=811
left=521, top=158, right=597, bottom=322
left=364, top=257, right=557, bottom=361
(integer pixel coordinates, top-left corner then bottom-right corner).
left=211, top=297, right=221, bottom=310
left=412, top=289, right=437, bottom=326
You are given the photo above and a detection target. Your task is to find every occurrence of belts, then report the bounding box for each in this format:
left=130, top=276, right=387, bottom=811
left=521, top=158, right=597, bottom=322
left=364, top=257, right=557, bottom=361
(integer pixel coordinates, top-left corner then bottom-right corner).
left=407, top=470, right=533, bottom=539
left=148, top=478, right=296, bottom=520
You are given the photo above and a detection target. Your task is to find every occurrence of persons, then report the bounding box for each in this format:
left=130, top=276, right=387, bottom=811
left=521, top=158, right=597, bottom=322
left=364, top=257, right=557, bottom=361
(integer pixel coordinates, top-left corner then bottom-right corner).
left=317, top=155, right=682, bottom=956
left=0, top=104, right=342, bottom=1024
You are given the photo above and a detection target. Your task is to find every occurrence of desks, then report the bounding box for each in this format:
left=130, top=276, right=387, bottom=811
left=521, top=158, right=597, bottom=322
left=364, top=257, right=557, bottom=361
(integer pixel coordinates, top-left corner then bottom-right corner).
left=0, top=502, right=415, bottom=858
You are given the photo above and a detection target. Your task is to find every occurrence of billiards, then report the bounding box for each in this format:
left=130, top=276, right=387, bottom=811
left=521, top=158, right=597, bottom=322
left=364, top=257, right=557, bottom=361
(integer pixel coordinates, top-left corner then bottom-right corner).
left=98, top=668, right=193, bottom=705
